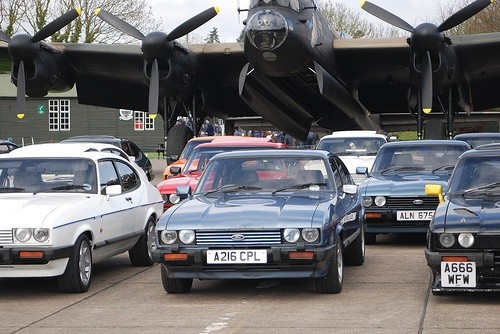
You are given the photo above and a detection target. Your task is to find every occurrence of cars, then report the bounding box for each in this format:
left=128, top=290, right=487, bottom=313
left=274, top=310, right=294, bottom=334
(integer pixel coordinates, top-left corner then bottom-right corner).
left=358, top=139, right=471, bottom=248
left=156, top=140, right=306, bottom=212
left=317, top=129, right=392, bottom=186
left=162, top=136, right=233, bottom=183
left=425, top=144, right=499, bottom=292
left=0, top=142, right=166, bottom=293
left=0, top=139, right=25, bottom=158
left=453, top=133, right=500, bottom=152
left=151, top=149, right=366, bottom=296
left=59, top=133, right=154, bottom=186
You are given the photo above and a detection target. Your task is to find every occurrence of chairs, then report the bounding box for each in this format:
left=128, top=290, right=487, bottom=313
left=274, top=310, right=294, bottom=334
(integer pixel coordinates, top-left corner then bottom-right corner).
left=228, top=170, right=258, bottom=185
left=294, top=170, right=327, bottom=191
left=14, top=170, right=42, bottom=188
left=440, top=155, right=460, bottom=168
left=467, top=166, right=500, bottom=190
left=392, top=154, right=414, bottom=167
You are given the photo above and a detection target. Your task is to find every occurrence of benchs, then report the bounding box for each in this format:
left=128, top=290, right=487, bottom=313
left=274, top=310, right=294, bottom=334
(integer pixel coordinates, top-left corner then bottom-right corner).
left=257, top=179, right=329, bottom=190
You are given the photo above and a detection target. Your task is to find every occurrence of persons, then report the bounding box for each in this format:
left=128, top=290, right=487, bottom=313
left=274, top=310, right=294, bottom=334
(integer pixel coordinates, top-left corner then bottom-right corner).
left=234, top=129, right=320, bottom=149
left=185, top=118, right=222, bottom=137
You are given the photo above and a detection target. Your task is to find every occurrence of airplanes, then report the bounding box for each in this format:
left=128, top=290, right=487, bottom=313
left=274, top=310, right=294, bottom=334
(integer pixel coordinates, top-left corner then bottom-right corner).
left=0, top=0, right=500, bottom=146
